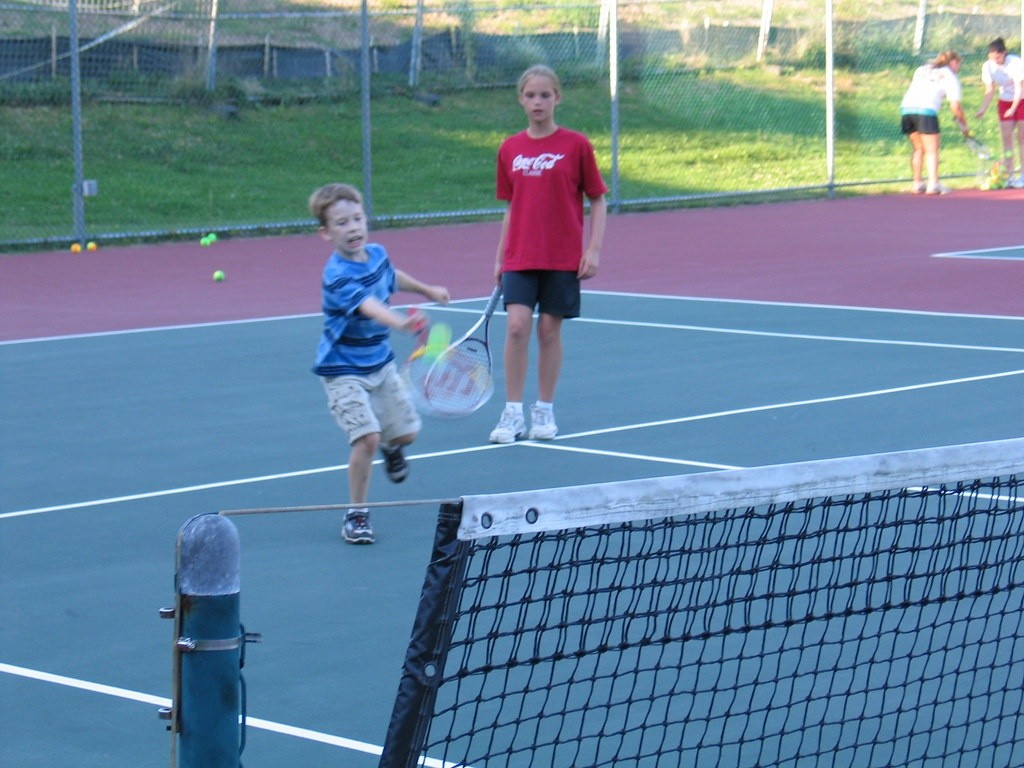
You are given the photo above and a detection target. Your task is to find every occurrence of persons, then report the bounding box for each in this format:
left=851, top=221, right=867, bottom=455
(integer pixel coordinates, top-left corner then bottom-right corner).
left=975, top=36, right=1024, bottom=188
left=491, top=63, right=608, bottom=444
left=309, top=183, right=451, bottom=545
left=901, top=50, right=969, bottom=195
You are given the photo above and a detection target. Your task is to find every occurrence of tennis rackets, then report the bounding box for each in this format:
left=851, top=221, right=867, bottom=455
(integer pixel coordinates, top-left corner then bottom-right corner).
left=953, top=116, right=990, bottom=160
left=404, top=306, right=495, bottom=416
left=423, top=282, right=505, bottom=416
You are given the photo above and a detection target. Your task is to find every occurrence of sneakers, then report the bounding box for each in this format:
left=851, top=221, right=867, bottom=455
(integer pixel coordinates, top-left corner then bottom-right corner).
left=341, top=508, right=376, bottom=544
left=381, top=443, right=410, bottom=483
left=490, top=407, right=527, bottom=444
left=529, top=405, right=558, bottom=439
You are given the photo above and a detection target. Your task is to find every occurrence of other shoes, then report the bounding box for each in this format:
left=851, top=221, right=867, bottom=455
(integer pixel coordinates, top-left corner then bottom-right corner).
left=927, top=184, right=950, bottom=195
left=912, top=183, right=925, bottom=194
left=1005, top=175, right=1024, bottom=188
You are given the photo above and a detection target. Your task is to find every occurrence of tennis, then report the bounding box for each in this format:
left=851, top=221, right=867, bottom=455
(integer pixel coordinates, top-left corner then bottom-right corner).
left=86, top=241, right=96, bottom=253
left=70, top=242, right=82, bottom=255
left=199, top=232, right=218, bottom=246
left=968, top=128, right=1013, bottom=190
left=213, top=269, right=225, bottom=282
